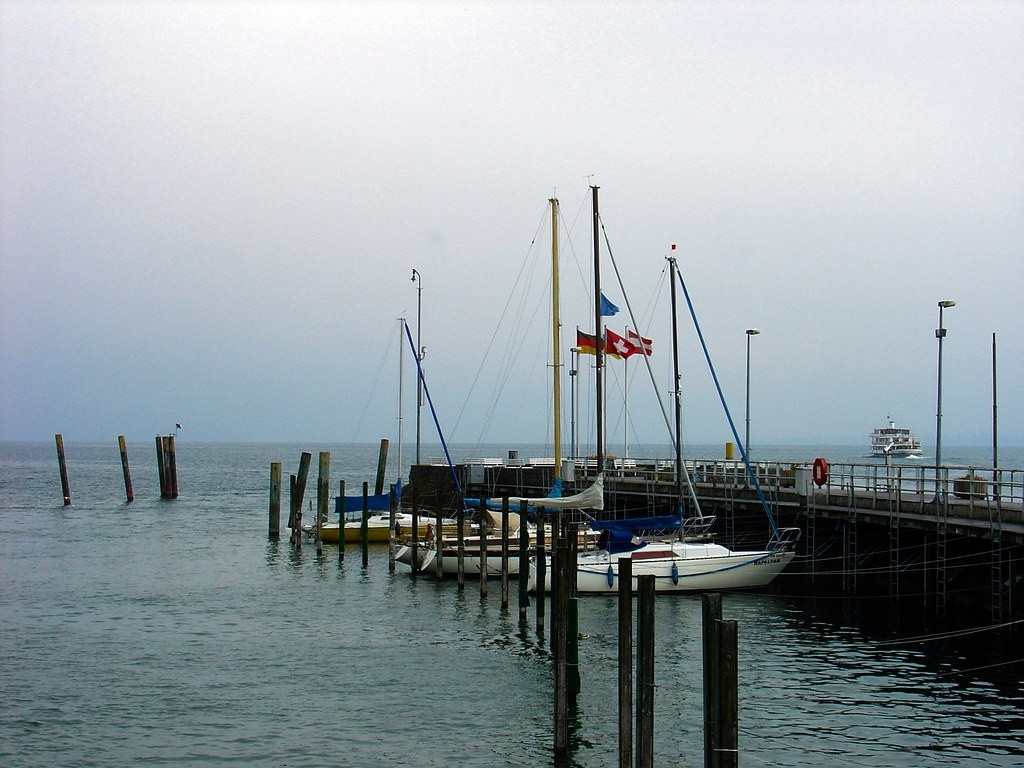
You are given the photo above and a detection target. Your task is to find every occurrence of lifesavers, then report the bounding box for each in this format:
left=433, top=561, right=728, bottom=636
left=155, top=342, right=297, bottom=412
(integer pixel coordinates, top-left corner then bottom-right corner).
left=812, top=458, right=828, bottom=486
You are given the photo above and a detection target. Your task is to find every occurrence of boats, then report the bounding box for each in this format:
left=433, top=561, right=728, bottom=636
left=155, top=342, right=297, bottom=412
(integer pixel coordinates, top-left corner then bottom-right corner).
left=869, top=416, right=922, bottom=455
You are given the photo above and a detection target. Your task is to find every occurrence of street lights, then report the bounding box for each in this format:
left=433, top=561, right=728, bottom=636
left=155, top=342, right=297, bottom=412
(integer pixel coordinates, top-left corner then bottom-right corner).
left=745, top=328, right=761, bottom=488
left=930, top=300, right=956, bottom=507
left=409, top=269, right=426, bottom=464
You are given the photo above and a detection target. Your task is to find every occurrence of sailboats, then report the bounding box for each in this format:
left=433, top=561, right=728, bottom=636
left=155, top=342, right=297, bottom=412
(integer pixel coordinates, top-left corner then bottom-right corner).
left=286, top=175, right=802, bottom=593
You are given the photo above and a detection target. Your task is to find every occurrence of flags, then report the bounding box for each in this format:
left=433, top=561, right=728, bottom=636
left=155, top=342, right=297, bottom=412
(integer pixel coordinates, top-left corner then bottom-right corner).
left=577, top=327, right=653, bottom=360
left=600, top=291, right=620, bottom=316
